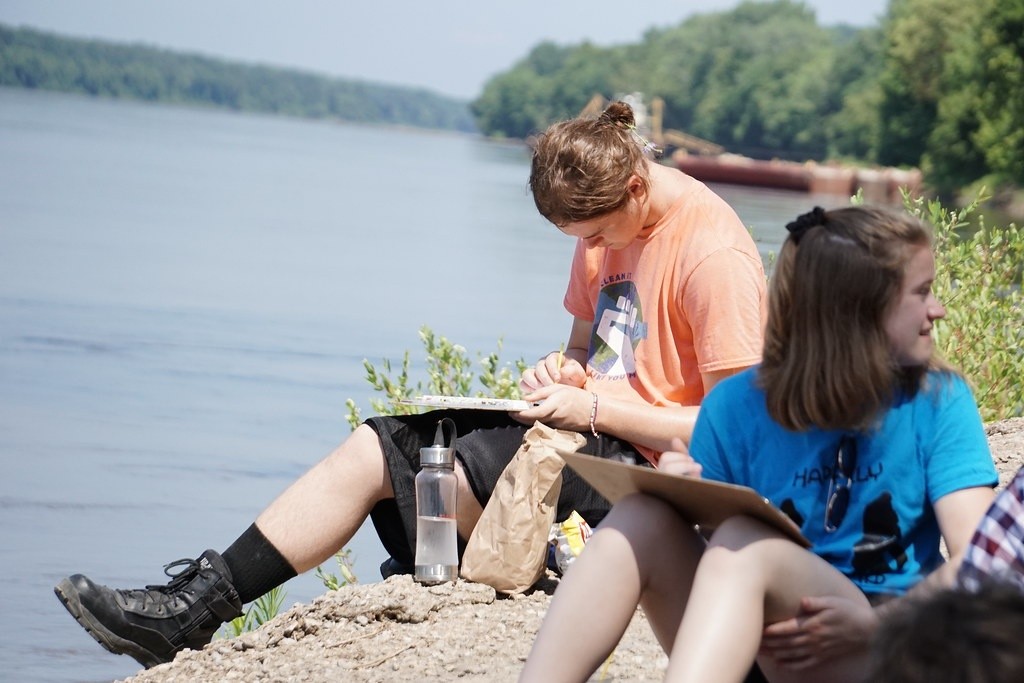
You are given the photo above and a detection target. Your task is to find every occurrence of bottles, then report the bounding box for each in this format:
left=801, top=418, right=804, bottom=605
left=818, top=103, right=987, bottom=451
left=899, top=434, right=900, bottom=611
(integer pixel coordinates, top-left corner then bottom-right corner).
left=414, top=419, right=458, bottom=585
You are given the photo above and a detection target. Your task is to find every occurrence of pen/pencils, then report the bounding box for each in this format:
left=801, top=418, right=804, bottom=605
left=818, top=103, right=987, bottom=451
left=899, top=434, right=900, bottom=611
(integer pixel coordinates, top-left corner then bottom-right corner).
left=554, top=341, right=565, bottom=384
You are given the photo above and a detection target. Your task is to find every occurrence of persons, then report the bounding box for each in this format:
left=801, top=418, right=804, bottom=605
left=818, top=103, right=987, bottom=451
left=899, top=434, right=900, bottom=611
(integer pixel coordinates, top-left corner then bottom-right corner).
left=517, top=204, right=999, bottom=683
left=54, top=100, right=768, bottom=670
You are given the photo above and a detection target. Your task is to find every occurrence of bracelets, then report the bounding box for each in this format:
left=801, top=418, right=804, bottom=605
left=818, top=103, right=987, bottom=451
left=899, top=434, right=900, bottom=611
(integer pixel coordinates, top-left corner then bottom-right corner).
left=591, top=392, right=599, bottom=439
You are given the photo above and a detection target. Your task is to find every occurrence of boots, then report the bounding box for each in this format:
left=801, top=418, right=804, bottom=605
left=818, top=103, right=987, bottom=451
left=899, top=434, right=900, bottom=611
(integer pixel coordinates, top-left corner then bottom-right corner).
left=55, top=549, right=246, bottom=670
